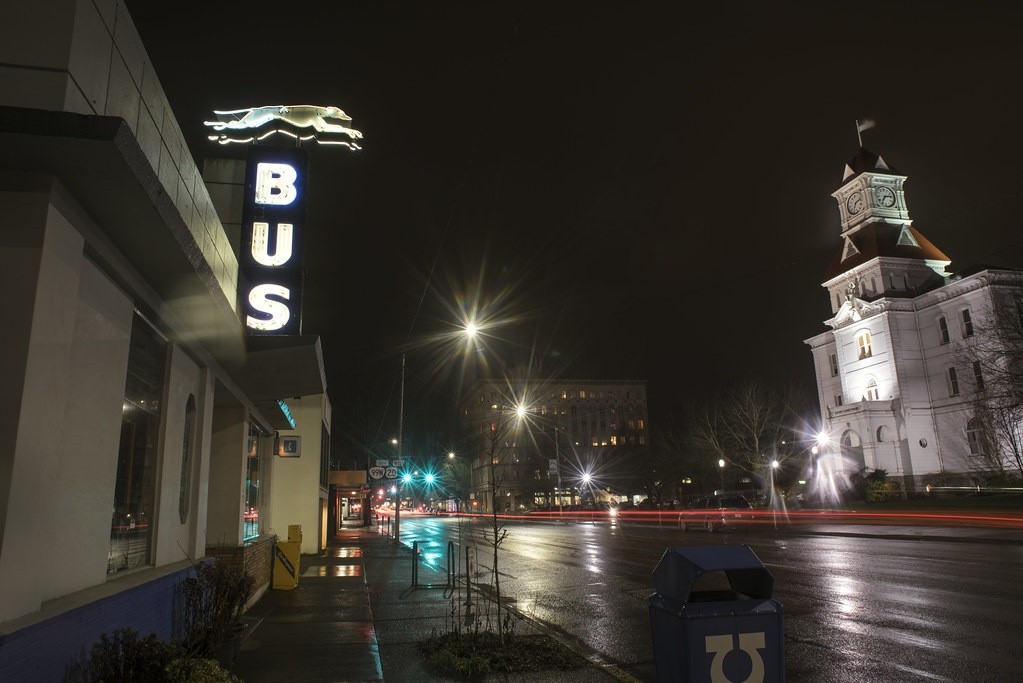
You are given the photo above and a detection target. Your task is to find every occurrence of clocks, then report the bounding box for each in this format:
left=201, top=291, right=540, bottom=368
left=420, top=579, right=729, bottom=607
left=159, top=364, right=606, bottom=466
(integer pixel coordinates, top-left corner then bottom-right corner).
left=829, top=172, right=913, bottom=239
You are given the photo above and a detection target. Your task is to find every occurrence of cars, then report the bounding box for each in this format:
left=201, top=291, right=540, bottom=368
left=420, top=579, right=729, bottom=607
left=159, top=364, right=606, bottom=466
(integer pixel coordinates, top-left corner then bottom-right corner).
left=677, top=493, right=755, bottom=534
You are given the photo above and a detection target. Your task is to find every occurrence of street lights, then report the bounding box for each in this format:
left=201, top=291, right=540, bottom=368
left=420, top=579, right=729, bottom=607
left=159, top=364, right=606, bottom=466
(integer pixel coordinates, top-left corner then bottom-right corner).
left=366, top=439, right=398, bottom=524
left=718, top=458, right=726, bottom=496
left=769, top=459, right=780, bottom=530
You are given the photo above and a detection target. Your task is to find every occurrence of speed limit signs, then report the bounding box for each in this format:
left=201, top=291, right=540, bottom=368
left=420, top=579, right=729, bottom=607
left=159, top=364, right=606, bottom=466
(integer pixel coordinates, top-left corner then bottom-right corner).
left=386, top=467, right=398, bottom=479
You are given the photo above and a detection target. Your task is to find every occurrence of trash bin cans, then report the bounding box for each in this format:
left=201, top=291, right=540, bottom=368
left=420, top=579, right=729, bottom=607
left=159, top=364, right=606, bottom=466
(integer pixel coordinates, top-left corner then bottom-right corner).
left=651, top=544, right=784, bottom=683
